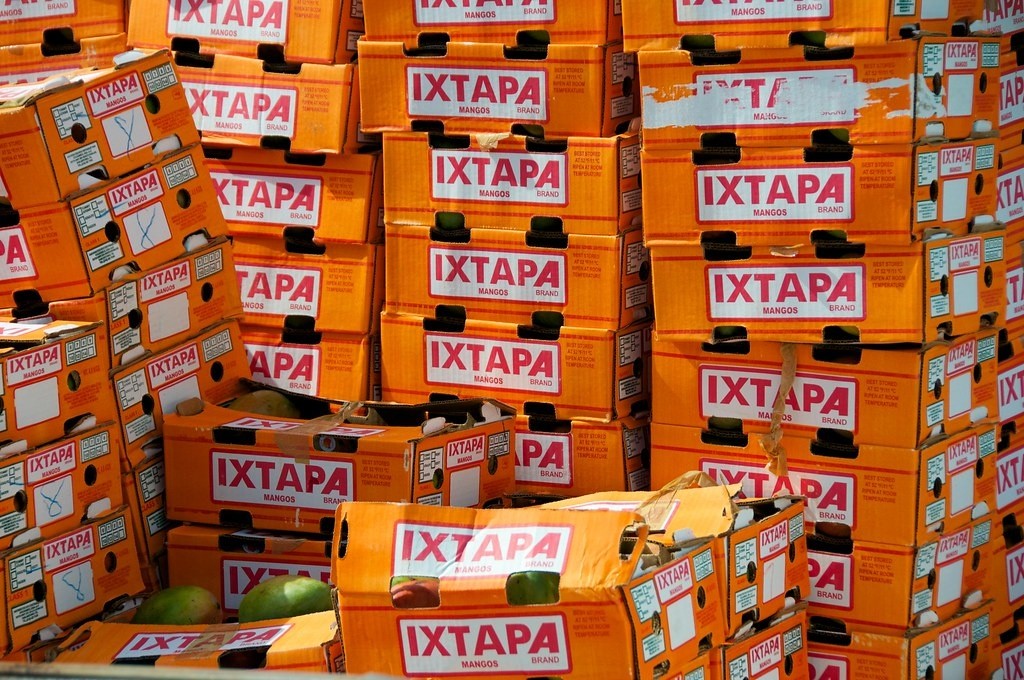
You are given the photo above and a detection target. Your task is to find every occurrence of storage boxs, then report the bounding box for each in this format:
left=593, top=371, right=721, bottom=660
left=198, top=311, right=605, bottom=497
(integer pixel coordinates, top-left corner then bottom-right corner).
left=50, top=610, right=346, bottom=676
left=126, top=0, right=365, bottom=66
left=0, top=589, right=162, bottom=663
left=0, top=31, right=127, bottom=85
left=0, top=503, right=146, bottom=659
left=108, top=318, right=253, bottom=470
left=0, top=319, right=113, bottom=449
left=0, top=143, right=229, bottom=309
left=131, top=47, right=382, bottom=156
left=203, top=146, right=385, bottom=246
left=134, top=457, right=180, bottom=563
left=0, top=0, right=128, bottom=46
left=0, top=47, right=200, bottom=211
left=0, top=234, right=245, bottom=369
left=161, top=376, right=517, bottom=535
left=329, top=0, right=1024, bottom=680
left=230, top=235, right=386, bottom=336
left=0, top=419, right=123, bottom=552
left=166, top=525, right=337, bottom=624
left=239, top=324, right=382, bottom=401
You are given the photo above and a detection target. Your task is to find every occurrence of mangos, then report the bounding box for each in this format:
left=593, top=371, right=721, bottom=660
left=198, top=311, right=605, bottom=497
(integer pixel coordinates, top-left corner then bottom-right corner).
left=127, top=585, right=224, bottom=624
left=391, top=571, right=562, bottom=608
left=225, top=390, right=295, bottom=418
left=238, top=574, right=333, bottom=624
